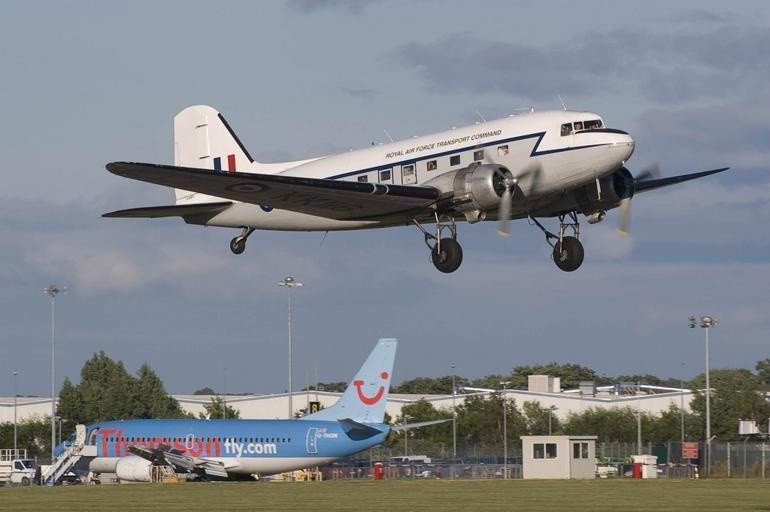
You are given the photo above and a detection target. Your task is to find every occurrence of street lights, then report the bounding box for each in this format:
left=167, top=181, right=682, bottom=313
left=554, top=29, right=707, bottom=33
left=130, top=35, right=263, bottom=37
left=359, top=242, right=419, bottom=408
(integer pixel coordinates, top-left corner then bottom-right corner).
left=42, top=285, right=68, bottom=464
left=544, top=404, right=558, bottom=436
left=501, top=382, right=511, bottom=477
left=403, top=415, right=409, bottom=455
left=450, top=364, right=456, bottom=455
left=279, top=278, right=303, bottom=419
left=689, top=316, right=718, bottom=477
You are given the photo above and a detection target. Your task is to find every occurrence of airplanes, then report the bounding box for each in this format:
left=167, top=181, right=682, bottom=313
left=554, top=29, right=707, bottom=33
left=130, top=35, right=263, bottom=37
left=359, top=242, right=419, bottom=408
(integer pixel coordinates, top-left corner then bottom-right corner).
left=54, top=338, right=454, bottom=480
left=102, top=103, right=731, bottom=273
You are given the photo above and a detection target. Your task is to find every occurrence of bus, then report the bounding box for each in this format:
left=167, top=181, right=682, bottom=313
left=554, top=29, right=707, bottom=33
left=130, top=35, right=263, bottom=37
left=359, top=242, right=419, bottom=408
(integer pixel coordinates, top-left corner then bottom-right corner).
left=388, top=456, right=431, bottom=478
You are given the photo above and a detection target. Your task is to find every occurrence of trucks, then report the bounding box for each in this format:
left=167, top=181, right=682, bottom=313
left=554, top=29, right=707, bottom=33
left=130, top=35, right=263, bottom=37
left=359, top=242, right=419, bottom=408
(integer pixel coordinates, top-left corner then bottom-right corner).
left=0, top=459, right=37, bottom=486
left=595, top=458, right=619, bottom=478
